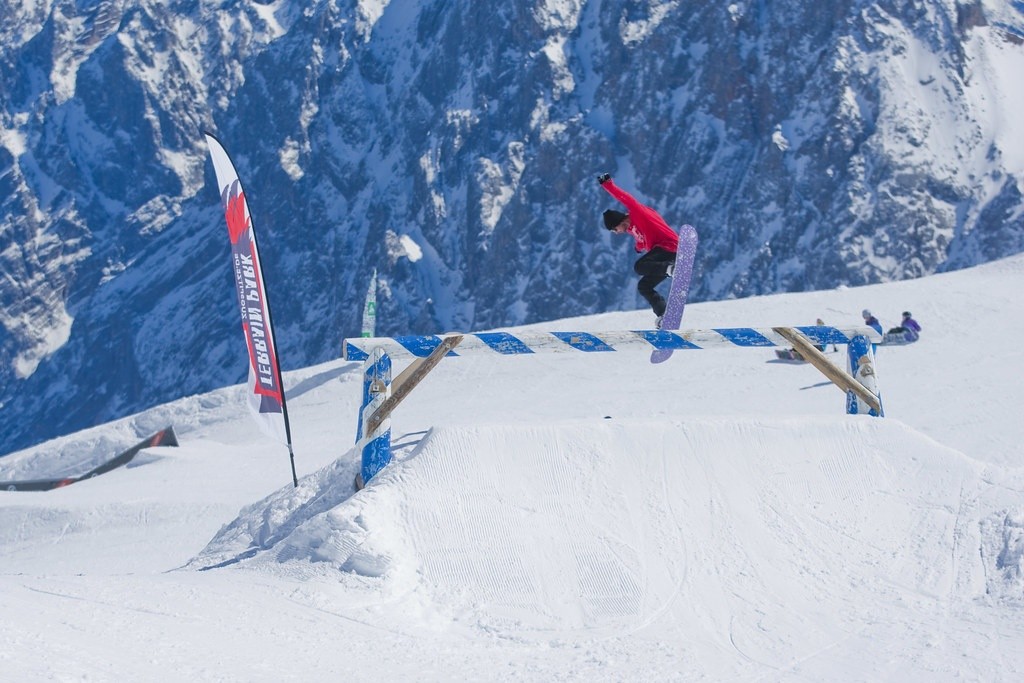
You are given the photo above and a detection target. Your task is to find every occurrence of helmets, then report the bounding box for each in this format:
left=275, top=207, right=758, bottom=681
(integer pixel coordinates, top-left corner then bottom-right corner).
left=902, top=311, right=910, bottom=317
left=863, top=310, right=870, bottom=315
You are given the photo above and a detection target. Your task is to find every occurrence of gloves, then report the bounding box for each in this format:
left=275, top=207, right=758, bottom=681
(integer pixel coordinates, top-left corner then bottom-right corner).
left=597, top=173, right=610, bottom=184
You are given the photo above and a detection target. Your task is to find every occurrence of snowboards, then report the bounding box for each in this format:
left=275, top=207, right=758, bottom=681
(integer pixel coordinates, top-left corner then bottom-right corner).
left=649, top=222, right=700, bottom=363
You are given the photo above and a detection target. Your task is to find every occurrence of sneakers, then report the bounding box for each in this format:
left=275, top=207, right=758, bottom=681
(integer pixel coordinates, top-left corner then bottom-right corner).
left=656, top=315, right=663, bottom=327
left=667, top=265, right=675, bottom=277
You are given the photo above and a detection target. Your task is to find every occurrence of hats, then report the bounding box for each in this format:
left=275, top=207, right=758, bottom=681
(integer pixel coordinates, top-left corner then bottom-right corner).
left=603, top=209, right=625, bottom=232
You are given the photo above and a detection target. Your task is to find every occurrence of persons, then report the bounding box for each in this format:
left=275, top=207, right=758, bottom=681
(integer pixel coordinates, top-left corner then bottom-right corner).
left=887, top=311, right=921, bottom=342
left=597, top=173, right=678, bottom=329
left=862, top=309, right=882, bottom=336
left=785, top=318, right=838, bottom=355
